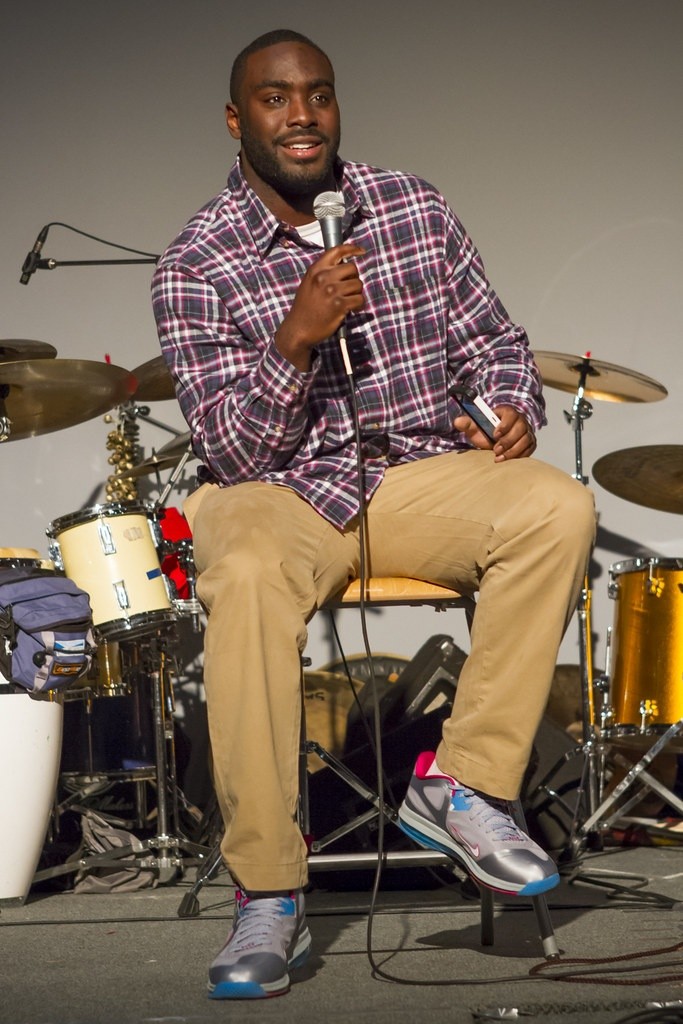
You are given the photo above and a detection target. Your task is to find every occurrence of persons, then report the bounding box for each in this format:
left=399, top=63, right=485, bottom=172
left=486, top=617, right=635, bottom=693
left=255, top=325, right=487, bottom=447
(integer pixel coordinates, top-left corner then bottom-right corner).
left=151, top=29, right=596, bottom=999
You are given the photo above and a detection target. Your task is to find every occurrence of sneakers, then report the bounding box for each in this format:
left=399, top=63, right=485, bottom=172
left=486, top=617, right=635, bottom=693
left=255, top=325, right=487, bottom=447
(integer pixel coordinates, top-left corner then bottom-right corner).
left=205, top=883, right=312, bottom=998
left=397, top=761, right=559, bottom=894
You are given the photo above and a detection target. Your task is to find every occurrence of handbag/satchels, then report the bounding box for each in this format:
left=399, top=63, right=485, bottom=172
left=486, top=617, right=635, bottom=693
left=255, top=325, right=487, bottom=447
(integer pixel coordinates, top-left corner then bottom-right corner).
left=1, top=567, right=98, bottom=693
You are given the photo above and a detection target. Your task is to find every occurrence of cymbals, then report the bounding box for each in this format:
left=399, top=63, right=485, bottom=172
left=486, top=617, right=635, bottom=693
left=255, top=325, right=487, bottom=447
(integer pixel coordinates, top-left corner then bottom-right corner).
left=1, top=338, right=58, bottom=361
left=129, top=354, right=177, bottom=402
left=528, top=349, right=669, bottom=405
left=0, top=358, right=138, bottom=443
left=590, top=443, right=683, bottom=519
left=118, top=451, right=194, bottom=479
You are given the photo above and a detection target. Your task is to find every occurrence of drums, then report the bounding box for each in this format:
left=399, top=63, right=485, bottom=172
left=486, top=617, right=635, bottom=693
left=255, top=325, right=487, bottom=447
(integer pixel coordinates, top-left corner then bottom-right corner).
left=1, top=546, right=65, bottom=908
left=603, top=555, right=682, bottom=762
left=63, top=638, right=126, bottom=701
left=42, top=503, right=183, bottom=640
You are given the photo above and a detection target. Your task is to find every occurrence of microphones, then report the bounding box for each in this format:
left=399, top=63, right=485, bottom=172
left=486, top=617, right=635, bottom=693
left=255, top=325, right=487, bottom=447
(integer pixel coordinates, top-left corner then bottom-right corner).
left=313, top=191, right=346, bottom=339
left=20, top=225, right=49, bottom=285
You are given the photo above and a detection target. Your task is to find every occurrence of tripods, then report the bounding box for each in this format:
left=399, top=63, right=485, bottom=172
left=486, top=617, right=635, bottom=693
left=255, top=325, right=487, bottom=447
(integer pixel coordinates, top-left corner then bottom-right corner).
left=526, top=371, right=683, bottom=852
left=30, top=636, right=226, bottom=883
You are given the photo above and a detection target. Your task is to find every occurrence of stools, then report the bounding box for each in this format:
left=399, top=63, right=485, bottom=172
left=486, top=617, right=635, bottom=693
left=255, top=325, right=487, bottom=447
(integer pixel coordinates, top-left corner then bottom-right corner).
left=295, top=574, right=563, bottom=957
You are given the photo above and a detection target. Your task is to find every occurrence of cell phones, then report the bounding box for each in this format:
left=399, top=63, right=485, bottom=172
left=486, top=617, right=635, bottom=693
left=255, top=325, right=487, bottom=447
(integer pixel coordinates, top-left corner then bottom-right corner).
left=448, top=384, right=502, bottom=445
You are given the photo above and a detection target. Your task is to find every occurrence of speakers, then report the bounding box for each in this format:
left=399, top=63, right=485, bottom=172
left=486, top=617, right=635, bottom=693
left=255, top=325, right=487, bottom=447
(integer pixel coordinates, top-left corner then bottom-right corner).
left=379, top=634, right=586, bottom=852
left=309, top=701, right=527, bottom=893
